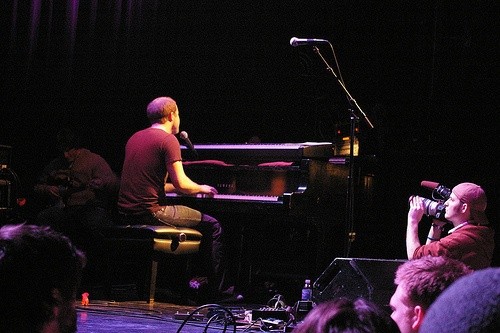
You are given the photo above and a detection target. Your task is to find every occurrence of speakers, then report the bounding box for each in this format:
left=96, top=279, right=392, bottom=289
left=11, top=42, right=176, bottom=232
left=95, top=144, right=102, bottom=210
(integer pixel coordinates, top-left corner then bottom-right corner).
left=314, top=258, right=407, bottom=307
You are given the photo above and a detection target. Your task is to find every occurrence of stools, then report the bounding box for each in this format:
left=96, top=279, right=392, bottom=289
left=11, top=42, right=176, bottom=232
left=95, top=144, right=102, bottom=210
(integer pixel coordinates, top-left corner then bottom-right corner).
left=71, top=224, right=203, bottom=310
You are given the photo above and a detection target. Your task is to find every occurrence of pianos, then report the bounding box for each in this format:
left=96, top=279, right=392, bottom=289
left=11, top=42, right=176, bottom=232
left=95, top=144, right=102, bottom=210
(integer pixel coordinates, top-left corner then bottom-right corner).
left=164, top=141, right=349, bottom=292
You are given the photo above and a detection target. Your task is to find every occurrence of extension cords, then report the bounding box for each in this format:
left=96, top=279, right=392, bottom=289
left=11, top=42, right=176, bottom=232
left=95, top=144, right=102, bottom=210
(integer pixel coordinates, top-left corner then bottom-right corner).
left=175, top=313, right=204, bottom=320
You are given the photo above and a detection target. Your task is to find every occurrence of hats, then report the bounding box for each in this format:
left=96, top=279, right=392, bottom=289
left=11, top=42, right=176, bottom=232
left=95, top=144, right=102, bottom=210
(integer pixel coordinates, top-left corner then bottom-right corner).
left=452, top=183, right=489, bottom=224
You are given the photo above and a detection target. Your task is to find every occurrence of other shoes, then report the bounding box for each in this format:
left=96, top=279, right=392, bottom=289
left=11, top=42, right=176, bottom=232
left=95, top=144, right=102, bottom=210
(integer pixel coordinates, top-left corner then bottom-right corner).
left=219, top=291, right=244, bottom=303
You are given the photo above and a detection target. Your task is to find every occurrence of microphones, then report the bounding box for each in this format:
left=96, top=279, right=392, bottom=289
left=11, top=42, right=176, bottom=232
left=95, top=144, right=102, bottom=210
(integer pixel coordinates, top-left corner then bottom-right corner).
left=290, top=37, right=328, bottom=47
left=180, top=131, right=198, bottom=156
left=421, top=181, right=440, bottom=191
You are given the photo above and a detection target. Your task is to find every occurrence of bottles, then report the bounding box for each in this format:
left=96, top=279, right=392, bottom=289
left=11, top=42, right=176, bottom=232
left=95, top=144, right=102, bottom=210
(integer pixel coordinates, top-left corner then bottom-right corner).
left=302, top=280, right=313, bottom=300
